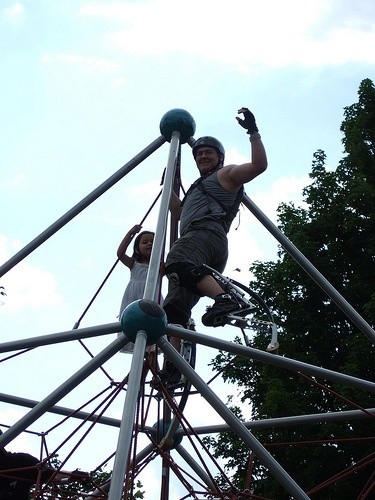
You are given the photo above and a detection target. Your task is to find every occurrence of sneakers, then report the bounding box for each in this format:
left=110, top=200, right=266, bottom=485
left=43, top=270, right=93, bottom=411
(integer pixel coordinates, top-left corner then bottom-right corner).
left=202, top=292, right=251, bottom=326
left=150, top=364, right=182, bottom=391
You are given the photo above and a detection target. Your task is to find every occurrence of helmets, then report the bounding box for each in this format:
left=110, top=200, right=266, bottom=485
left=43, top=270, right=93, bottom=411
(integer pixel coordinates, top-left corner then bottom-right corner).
left=192, top=136, right=225, bottom=159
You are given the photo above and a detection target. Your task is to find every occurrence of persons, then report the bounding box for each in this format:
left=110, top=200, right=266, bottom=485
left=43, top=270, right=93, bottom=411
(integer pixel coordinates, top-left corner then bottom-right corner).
left=117, top=225, right=165, bottom=384
left=147, top=107, right=268, bottom=394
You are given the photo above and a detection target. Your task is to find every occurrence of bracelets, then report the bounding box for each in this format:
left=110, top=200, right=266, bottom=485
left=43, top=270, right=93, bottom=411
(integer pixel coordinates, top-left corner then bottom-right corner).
left=249, top=132, right=262, bottom=143
